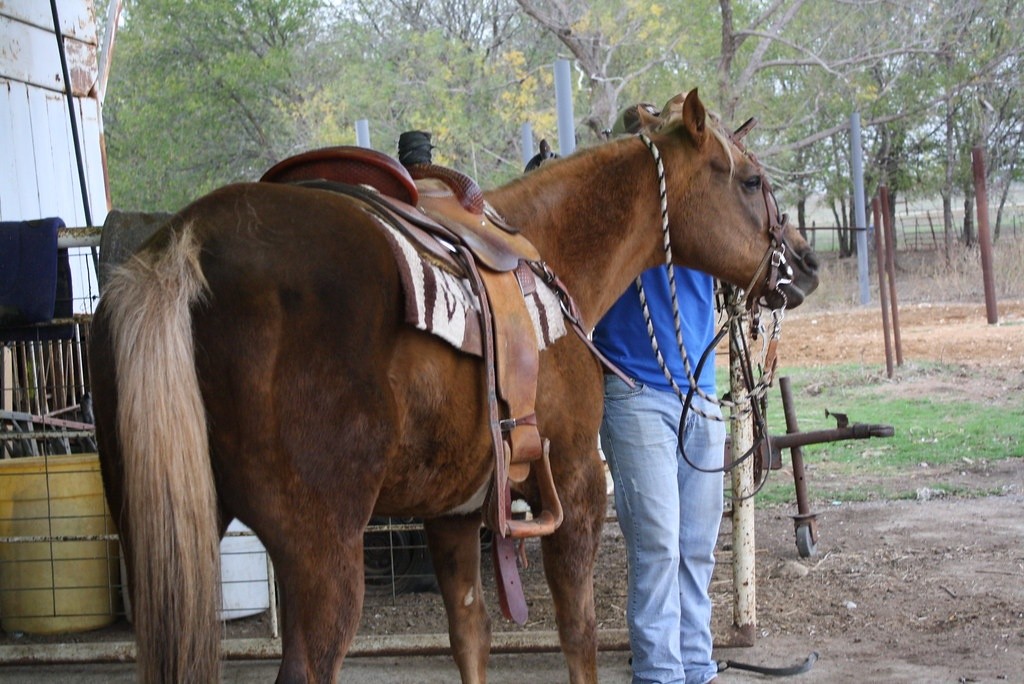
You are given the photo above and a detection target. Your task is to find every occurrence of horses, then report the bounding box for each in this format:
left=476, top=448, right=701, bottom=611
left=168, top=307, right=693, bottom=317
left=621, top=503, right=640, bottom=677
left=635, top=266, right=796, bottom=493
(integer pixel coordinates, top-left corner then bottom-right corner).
left=85, top=86, right=821, bottom=684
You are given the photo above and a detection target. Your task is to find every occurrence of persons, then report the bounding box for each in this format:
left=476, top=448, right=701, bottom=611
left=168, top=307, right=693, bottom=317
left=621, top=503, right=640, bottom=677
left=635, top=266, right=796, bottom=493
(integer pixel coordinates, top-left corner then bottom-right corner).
left=590, top=100, right=731, bottom=684
